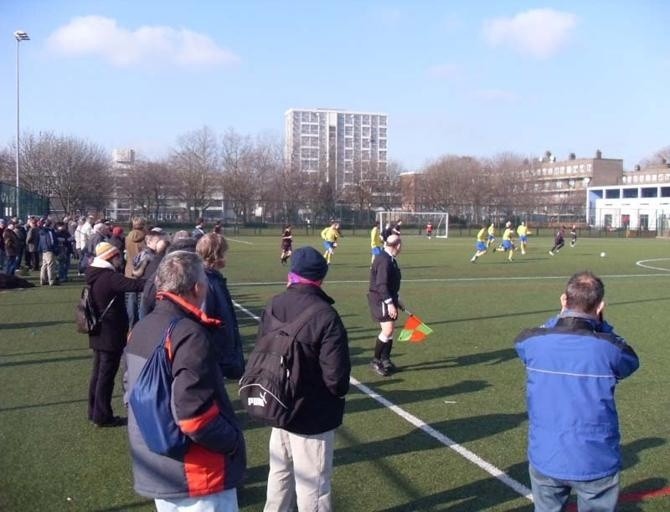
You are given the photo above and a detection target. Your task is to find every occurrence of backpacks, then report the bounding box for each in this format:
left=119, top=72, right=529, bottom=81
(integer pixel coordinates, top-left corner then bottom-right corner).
left=76, top=276, right=117, bottom=334
left=239, top=293, right=330, bottom=428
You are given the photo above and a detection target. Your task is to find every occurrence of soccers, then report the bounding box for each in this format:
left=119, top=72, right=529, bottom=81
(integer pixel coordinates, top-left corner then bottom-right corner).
left=601, top=252, right=605, bottom=257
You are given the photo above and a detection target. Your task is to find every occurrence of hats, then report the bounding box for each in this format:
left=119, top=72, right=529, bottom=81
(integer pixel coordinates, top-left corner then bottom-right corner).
left=290, top=247, right=328, bottom=280
left=96, top=242, right=120, bottom=260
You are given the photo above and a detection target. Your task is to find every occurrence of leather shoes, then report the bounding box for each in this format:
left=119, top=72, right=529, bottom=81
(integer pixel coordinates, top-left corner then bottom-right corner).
left=95, top=416, right=121, bottom=428
left=90, top=416, right=114, bottom=424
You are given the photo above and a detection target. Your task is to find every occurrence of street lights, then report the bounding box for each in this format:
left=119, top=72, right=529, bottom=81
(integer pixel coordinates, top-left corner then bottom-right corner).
left=13, top=30, right=32, bottom=218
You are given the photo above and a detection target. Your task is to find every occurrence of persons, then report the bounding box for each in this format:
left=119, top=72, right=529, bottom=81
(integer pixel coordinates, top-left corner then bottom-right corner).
left=487, top=215, right=520, bottom=227
left=470, top=224, right=487, bottom=263
left=517, top=220, right=531, bottom=254
left=367, top=234, right=406, bottom=378
left=194, top=234, right=244, bottom=380
left=370, top=221, right=382, bottom=270
left=380, top=229, right=391, bottom=246
left=569, top=224, right=578, bottom=247
left=191, top=217, right=206, bottom=244
left=426, top=222, right=433, bottom=239
left=333, top=222, right=343, bottom=253
left=513, top=271, right=639, bottom=512
left=119, top=251, right=245, bottom=512
left=320, top=221, right=339, bottom=264
left=279, top=225, right=292, bottom=264
left=212, top=220, right=224, bottom=234
left=406, top=214, right=446, bottom=223
left=493, top=221, right=514, bottom=262
left=242, top=246, right=351, bottom=512
left=0, top=215, right=195, bottom=285
left=81, top=242, right=145, bottom=426
left=487, top=222, right=496, bottom=255
left=549, top=223, right=566, bottom=256
left=392, top=219, right=402, bottom=235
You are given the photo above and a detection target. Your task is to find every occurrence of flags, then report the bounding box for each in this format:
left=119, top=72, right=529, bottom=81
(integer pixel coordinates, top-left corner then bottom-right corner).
left=397, top=314, right=433, bottom=342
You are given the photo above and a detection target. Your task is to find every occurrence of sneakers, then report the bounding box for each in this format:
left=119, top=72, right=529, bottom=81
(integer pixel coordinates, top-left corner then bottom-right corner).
left=372, top=362, right=386, bottom=377
left=384, top=360, right=395, bottom=370
